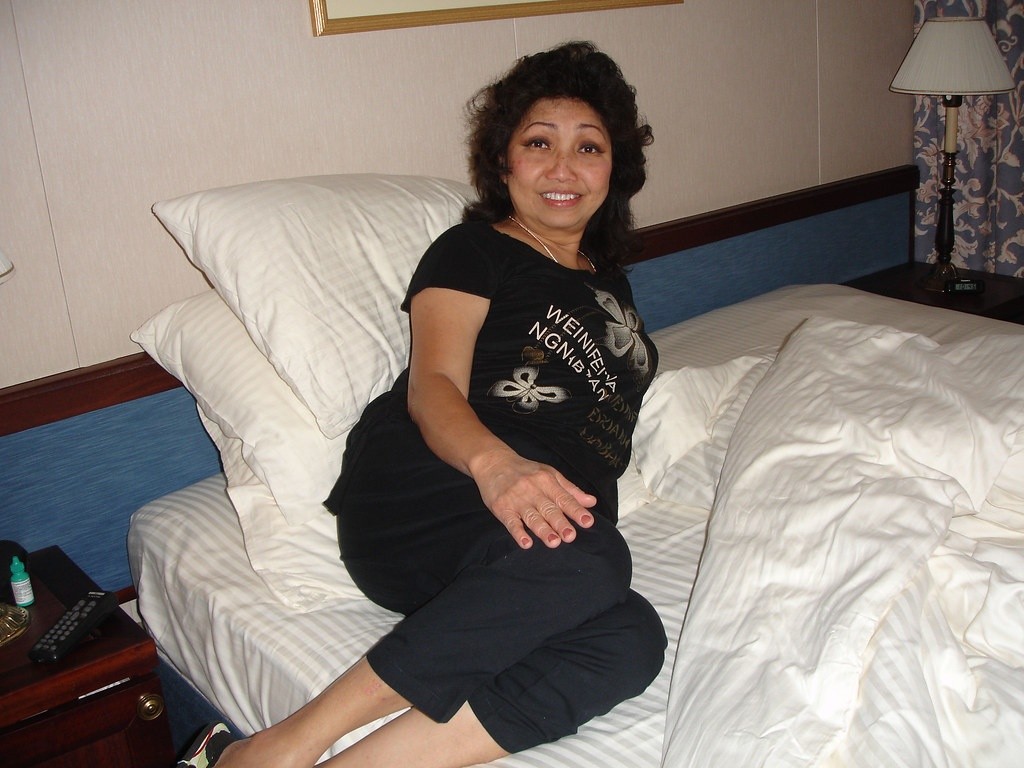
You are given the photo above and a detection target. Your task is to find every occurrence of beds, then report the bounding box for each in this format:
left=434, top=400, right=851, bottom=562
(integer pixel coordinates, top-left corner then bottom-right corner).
left=1, top=163, right=1024, bottom=767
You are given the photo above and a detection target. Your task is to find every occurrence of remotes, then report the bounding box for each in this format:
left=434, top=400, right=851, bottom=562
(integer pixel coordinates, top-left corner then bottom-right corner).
left=29, top=590, right=119, bottom=662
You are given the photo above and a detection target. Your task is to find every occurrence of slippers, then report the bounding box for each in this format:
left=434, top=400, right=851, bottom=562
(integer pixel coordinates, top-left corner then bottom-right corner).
left=175, top=720, right=235, bottom=768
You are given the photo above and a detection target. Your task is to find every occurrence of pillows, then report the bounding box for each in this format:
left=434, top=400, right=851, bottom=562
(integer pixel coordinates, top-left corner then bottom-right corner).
left=129, top=289, right=341, bottom=539
left=195, top=402, right=375, bottom=618
left=150, top=169, right=481, bottom=442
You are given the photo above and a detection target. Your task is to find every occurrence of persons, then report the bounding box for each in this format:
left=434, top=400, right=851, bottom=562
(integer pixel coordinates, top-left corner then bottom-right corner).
left=182, top=42, right=669, bottom=767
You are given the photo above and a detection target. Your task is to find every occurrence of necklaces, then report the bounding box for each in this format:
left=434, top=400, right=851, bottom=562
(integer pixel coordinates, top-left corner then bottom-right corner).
left=509, top=216, right=596, bottom=272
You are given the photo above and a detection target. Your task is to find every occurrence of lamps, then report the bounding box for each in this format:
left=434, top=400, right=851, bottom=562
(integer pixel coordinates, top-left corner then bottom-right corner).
left=889, top=14, right=1015, bottom=296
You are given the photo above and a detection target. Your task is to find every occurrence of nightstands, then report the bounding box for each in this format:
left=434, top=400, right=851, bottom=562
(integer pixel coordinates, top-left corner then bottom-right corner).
left=1, top=544, right=177, bottom=768
left=838, top=263, right=1024, bottom=329
